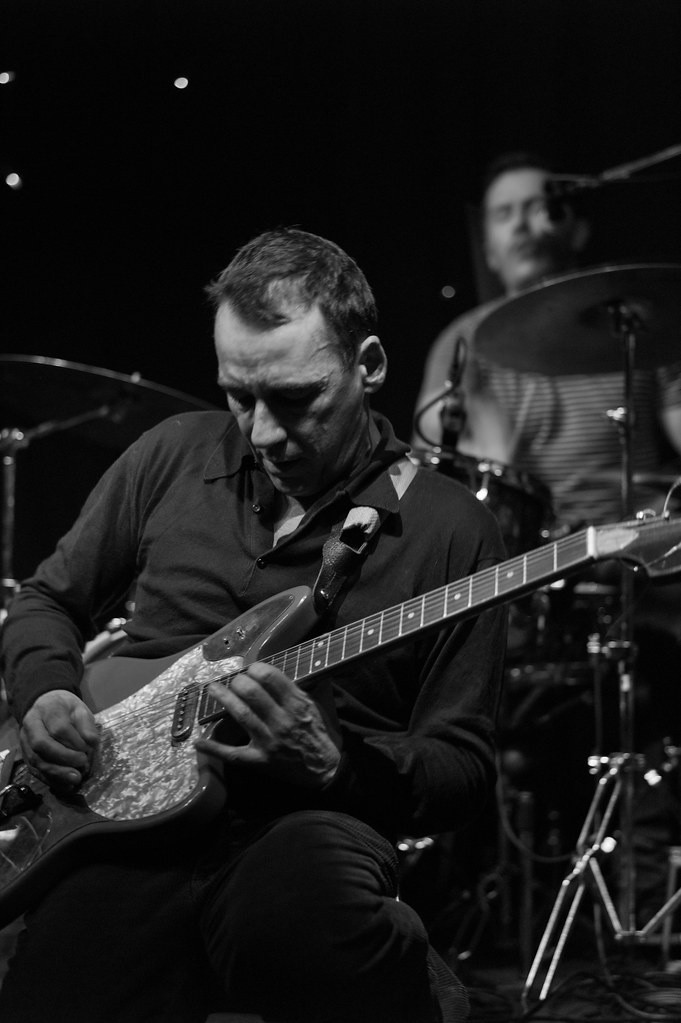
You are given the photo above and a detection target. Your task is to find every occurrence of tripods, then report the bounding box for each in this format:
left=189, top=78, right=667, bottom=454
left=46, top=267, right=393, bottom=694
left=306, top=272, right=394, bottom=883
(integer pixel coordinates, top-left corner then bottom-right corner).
left=515, top=326, right=681, bottom=1023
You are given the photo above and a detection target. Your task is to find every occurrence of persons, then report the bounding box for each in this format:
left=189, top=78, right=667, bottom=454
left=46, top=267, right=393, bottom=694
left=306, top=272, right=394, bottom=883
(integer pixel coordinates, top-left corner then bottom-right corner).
left=0, top=231, right=510, bottom=1023
left=405, top=170, right=681, bottom=946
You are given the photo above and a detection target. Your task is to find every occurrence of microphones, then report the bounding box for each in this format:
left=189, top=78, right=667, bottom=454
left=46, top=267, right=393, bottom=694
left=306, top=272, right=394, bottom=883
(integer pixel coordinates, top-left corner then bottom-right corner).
left=442, top=358, right=467, bottom=454
left=538, top=175, right=572, bottom=235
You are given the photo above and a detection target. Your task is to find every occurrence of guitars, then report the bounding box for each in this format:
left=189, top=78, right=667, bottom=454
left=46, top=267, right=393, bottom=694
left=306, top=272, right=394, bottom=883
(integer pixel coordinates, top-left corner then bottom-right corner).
left=0, top=508, right=681, bottom=933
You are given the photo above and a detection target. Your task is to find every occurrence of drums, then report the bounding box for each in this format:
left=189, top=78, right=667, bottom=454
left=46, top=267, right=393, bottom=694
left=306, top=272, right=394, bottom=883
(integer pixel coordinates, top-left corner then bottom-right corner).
left=507, top=469, right=681, bottom=974
left=405, top=446, right=558, bottom=651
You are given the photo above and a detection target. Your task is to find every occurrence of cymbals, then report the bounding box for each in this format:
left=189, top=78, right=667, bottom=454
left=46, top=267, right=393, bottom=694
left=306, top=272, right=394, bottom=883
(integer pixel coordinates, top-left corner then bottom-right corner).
left=473, top=264, right=681, bottom=378
left=0, top=352, right=226, bottom=439
